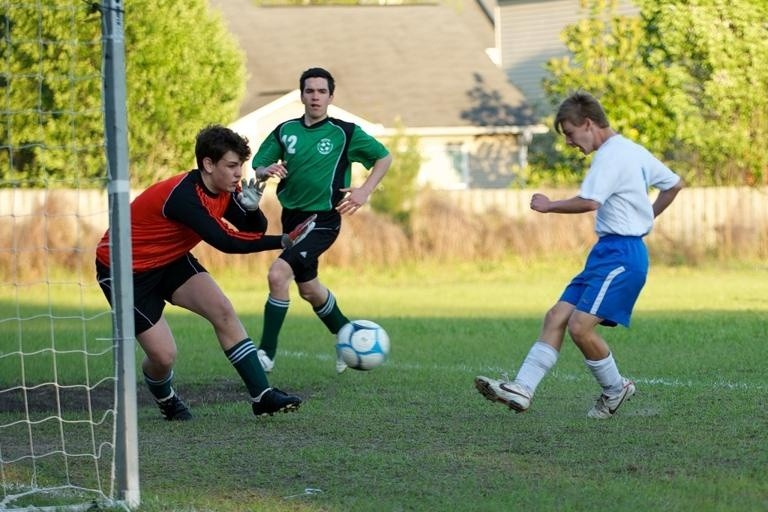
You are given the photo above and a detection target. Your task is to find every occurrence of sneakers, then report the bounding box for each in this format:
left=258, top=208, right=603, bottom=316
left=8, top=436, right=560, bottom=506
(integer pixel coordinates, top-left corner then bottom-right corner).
left=475, top=376, right=530, bottom=414
left=250, top=387, right=302, bottom=417
left=587, top=378, right=635, bottom=420
left=257, top=350, right=275, bottom=373
left=154, top=387, right=192, bottom=421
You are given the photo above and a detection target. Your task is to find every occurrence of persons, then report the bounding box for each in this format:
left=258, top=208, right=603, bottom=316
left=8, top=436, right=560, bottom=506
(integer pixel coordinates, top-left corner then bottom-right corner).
left=475, top=93, right=681, bottom=420
left=251, top=67, right=391, bottom=375
left=95, top=126, right=319, bottom=422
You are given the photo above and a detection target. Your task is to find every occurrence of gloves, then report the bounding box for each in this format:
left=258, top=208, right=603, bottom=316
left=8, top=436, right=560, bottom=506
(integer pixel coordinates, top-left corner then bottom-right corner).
left=238, top=179, right=265, bottom=211
left=282, top=214, right=318, bottom=248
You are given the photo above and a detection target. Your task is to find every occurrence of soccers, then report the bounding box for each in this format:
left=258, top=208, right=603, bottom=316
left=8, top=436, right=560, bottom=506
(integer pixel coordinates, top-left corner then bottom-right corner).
left=337, top=319, right=390, bottom=369
